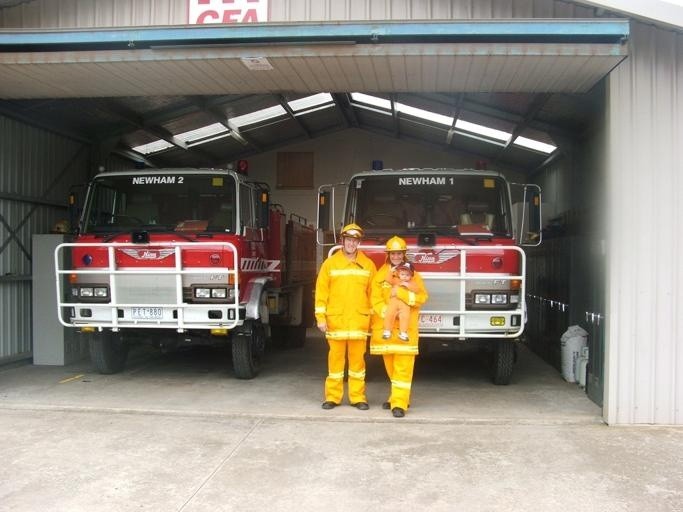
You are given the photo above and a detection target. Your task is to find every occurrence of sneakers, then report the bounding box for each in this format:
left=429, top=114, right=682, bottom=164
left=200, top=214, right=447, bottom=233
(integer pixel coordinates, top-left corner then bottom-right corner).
left=392, top=407, right=404, bottom=417
left=350, top=402, right=368, bottom=410
left=382, top=331, right=391, bottom=339
left=397, top=331, right=408, bottom=341
left=322, top=402, right=335, bottom=409
left=382, top=403, right=408, bottom=409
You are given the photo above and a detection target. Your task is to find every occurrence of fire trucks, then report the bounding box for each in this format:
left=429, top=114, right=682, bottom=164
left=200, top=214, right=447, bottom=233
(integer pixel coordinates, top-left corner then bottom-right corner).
left=315, top=158, right=546, bottom=389
left=53, top=164, right=328, bottom=377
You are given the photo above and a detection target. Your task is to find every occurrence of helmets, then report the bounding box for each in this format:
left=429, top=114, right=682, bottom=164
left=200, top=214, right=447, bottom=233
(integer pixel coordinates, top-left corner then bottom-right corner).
left=341, top=223, right=364, bottom=238
left=384, top=235, right=408, bottom=251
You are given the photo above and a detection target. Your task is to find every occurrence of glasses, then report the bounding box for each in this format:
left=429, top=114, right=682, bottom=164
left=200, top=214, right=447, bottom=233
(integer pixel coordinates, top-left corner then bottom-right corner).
left=342, top=229, right=364, bottom=238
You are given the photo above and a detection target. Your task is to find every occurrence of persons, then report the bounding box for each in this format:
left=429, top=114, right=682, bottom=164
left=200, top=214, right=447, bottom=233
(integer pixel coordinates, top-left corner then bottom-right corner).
left=314, top=223, right=377, bottom=409
left=370, top=236, right=430, bottom=418
left=381, top=262, right=418, bottom=341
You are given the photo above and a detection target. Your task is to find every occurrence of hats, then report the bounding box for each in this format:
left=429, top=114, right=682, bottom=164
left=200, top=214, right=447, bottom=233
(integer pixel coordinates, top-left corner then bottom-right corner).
left=397, top=262, right=414, bottom=276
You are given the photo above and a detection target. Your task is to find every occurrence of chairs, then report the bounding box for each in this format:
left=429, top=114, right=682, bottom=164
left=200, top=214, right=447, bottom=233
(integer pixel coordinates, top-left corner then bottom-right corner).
left=125, top=192, right=232, bottom=232
left=362, top=193, right=502, bottom=233
